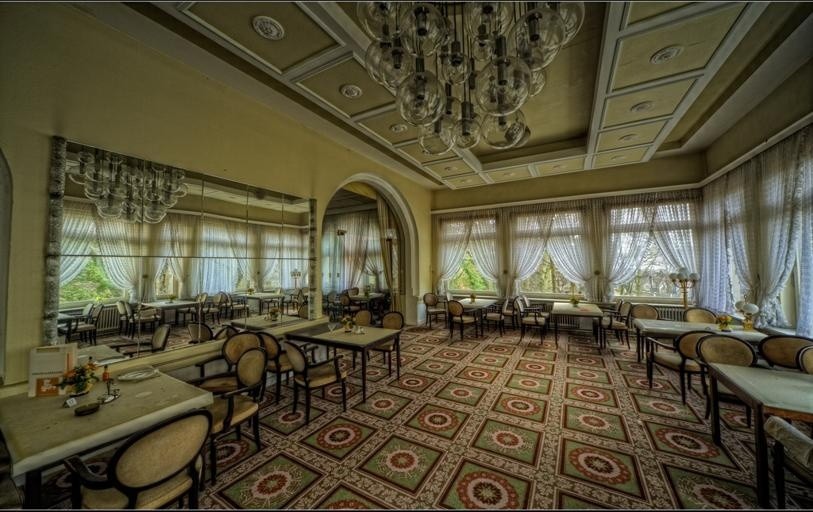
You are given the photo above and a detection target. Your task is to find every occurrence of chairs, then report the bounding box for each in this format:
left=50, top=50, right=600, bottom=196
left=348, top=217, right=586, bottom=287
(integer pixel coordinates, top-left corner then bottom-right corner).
left=631, top=305, right=659, bottom=359
left=57, top=303, right=94, bottom=342
left=348, top=288, right=368, bottom=310
left=175, top=292, right=207, bottom=327
left=123, top=302, right=161, bottom=340
left=327, top=289, right=344, bottom=319
left=646, top=331, right=716, bottom=406
left=258, top=330, right=312, bottom=404
left=797, top=345, right=813, bottom=375
left=503, top=296, right=519, bottom=331
left=598, top=302, right=632, bottom=349
left=194, top=331, right=262, bottom=427
left=262, top=288, right=282, bottom=313
left=483, top=298, right=508, bottom=336
left=302, top=287, right=308, bottom=300
left=684, top=308, right=718, bottom=324
left=593, top=299, right=622, bottom=347
left=201, top=291, right=227, bottom=324
left=116, top=299, right=140, bottom=336
left=339, top=293, right=363, bottom=319
left=282, top=287, right=300, bottom=315
left=63, top=409, right=213, bottom=512
left=424, top=293, right=447, bottom=330
left=228, top=293, right=250, bottom=320
left=334, top=289, right=348, bottom=314
left=219, top=292, right=232, bottom=319
left=368, top=297, right=387, bottom=324
left=59, top=304, right=105, bottom=346
left=758, top=335, right=812, bottom=371
left=208, top=347, right=267, bottom=485
left=522, top=294, right=551, bottom=335
left=763, top=415, right=813, bottom=509
left=284, top=341, right=347, bottom=425
left=371, top=311, right=405, bottom=377
left=517, top=300, right=546, bottom=345
left=333, top=310, right=371, bottom=370
left=446, top=299, right=479, bottom=339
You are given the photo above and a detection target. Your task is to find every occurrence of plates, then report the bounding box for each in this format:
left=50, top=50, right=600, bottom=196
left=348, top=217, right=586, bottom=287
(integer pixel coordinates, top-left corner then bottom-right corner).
left=118, top=368, right=157, bottom=381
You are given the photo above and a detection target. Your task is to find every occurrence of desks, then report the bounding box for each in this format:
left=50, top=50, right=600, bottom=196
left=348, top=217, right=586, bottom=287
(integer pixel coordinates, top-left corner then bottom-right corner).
left=58, top=313, right=76, bottom=343
left=552, top=302, right=604, bottom=350
left=705, top=364, right=813, bottom=507
left=140, top=301, right=200, bottom=325
left=231, top=314, right=301, bottom=334
left=350, top=293, right=387, bottom=312
left=0, top=366, right=214, bottom=512
left=80, top=346, right=129, bottom=367
left=634, top=318, right=769, bottom=382
left=458, top=299, right=500, bottom=337
left=239, top=293, right=285, bottom=315
left=286, top=322, right=402, bottom=403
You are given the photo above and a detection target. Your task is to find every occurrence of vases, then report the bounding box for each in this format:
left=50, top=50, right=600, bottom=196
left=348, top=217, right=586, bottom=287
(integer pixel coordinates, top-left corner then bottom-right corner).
left=68, top=384, right=93, bottom=397
left=720, top=323, right=732, bottom=332
left=344, top=324, right=351, bottom=332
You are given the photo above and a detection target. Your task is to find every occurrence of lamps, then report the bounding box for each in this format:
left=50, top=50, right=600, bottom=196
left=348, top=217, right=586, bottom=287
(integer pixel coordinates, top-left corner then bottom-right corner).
left=68, top=147, right=184, bottom=223
left=384, top=225, right=398, bottom=241
left=736, top=301, right=758, bottom=331
left=357, top=0, right=585, bottom=154
left=670, top=268, right=701, bottom=310
left=291, top=269, right=303, bottom=288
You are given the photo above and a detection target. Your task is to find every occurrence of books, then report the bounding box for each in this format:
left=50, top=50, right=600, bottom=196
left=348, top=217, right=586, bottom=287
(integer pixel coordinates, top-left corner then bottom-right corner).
left=28, top=341, right=79, bottom=399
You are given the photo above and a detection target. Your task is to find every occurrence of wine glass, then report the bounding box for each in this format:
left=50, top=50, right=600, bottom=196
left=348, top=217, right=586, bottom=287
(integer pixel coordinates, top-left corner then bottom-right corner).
left=328, top=324, right=336, bottom=336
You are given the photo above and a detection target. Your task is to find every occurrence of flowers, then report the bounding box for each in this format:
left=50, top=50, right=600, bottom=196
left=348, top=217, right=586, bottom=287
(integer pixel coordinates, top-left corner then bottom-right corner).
left=55, top=363, right=99, bottom=393
left=717, top=315, right=731, bottom=326
left=340, top=315, right=357, bottom=329
